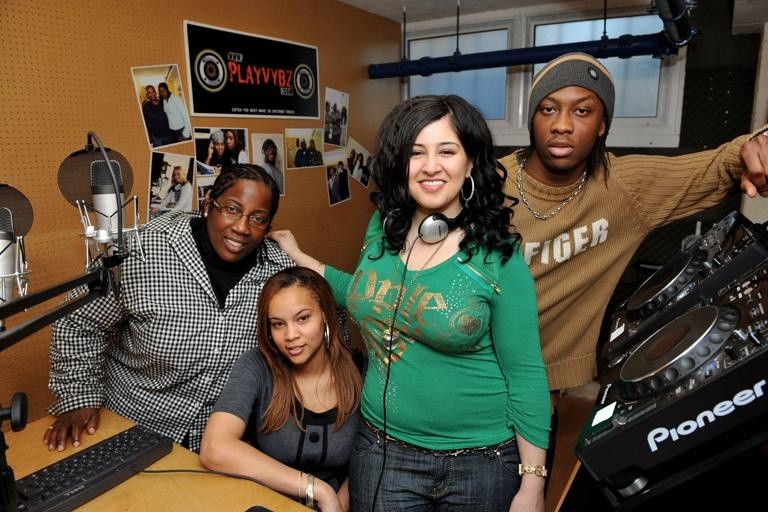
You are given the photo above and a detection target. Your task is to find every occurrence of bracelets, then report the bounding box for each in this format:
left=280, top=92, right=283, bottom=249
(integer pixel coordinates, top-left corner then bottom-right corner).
left=306, top=472, right=314, bottom=510
left=298, top=470, right=303, bottom=499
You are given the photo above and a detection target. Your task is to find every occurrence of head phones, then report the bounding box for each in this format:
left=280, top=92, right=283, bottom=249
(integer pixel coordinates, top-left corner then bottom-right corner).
left=382, top=201, right=471, bottom=244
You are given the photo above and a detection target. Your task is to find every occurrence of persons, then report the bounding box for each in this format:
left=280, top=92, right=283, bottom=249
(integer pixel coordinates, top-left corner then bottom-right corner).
left=294, top=139, right=309, bottom=166
left=208, top=129, right=232, bottom=166
left=307, top=139, right=323, bottom=166
left=151, top=166, right=193, bottom=215
left=325, top=100, right=331, bottom=143
left=328, top=164, right=336, bottom=193
left=346, top=149, right=373, bottom=187
left=42, top=164, right=350, bottom=455
left=199, top=267, right=362, bottom=512
left=225, top=130, right=249, bottom=164
left=330, top=103, right=342, bottom=145
left=337, top=161, right=349, bottom=199
left=494, top=52, right=768, bottom=500
left=158, top=83, right=192, bottom=141
left=258, top=139, right=284, bottom=195
left=142, top=85, right=168, bottom=147
left=266, top=94, right=551, bottom=512
left=326, top=163, right=336, bottom=179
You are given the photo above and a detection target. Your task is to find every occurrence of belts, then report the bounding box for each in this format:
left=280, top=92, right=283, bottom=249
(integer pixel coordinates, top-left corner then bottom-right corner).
left=360, top=415, right=516, bottom=457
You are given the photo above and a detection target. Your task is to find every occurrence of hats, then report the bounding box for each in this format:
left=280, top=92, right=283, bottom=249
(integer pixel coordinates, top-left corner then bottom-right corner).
left=262, top=139, right=276, bottom=150
left=210, top=130, right=224, bottom=143
left=528, top=52, right=615, bottom=130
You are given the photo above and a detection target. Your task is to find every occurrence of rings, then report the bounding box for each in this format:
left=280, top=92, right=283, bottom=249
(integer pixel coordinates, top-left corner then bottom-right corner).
left=49, top=425, right=55, bottom=431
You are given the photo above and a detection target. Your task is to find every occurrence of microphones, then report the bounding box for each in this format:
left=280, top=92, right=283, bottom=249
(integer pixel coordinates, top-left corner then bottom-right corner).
left=0, top=208, right=13, bottom=306
left=91, top=161, right=124, bottom=240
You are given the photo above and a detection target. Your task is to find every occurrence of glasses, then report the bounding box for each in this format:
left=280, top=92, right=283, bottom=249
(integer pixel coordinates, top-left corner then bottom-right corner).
left=209, top=197, right=272, bottom=231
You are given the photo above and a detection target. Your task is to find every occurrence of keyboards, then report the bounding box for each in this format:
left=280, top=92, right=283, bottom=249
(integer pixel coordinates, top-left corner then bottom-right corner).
left=15, top=425, right=175, bottom=512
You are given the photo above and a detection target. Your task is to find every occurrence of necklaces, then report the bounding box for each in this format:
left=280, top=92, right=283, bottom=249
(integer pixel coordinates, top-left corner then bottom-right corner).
left=377, top=218, right=449, bottom=351
left=517, top=151, right=589, bottom=221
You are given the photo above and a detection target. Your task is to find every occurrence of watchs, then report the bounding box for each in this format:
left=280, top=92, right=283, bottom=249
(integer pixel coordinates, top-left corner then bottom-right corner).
left=519, top=462, right=548, bottom=479
